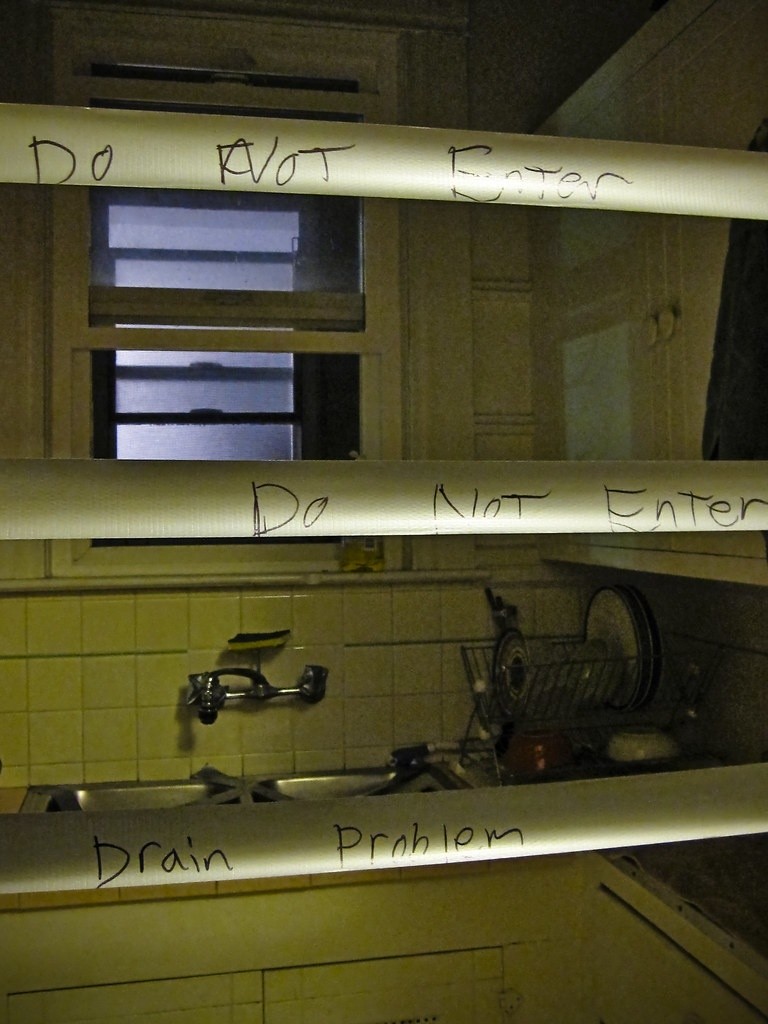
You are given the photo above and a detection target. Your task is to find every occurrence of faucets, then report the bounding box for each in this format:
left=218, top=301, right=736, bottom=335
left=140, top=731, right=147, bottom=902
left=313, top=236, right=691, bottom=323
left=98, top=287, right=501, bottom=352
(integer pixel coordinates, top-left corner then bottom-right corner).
left=187, top=662, right=277, bottom=725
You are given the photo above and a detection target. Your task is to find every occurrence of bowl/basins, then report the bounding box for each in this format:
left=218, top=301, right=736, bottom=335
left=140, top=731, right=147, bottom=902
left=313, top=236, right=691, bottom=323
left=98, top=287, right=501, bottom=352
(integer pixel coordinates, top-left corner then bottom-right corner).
left=608, top=726, right=681, bottom=761
left=509, top=730, right=574, bottom=770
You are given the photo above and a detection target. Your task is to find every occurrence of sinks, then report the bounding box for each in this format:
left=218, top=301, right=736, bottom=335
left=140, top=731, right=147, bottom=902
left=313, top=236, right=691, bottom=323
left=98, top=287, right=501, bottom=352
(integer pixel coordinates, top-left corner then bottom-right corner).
left=238, top=760, right=475, bottom=808
left=16, top=776, right=256, bottom=816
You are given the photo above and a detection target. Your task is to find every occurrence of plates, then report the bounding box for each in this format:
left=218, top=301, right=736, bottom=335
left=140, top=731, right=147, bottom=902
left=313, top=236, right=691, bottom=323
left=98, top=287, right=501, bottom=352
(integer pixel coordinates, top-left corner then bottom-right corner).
left=585, top=583, right=666, bottom=712
left=622, top=677, right=623, bottom=678
left=493, top=626, right=531, bottom=720
